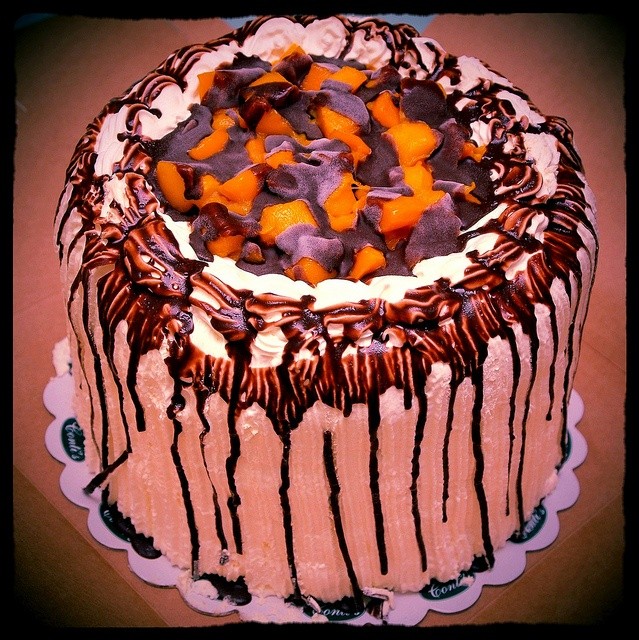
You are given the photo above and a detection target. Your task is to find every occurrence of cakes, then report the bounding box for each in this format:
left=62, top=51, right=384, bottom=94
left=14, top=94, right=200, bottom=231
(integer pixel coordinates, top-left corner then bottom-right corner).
left=53, top=16, right=600, bottom=618
